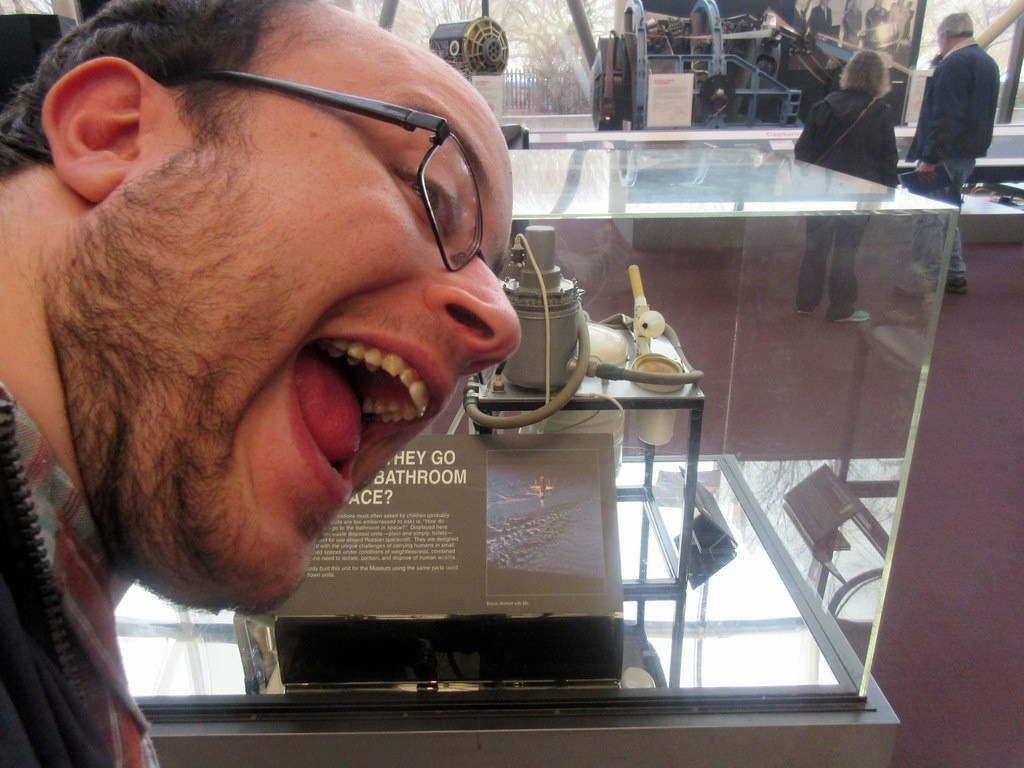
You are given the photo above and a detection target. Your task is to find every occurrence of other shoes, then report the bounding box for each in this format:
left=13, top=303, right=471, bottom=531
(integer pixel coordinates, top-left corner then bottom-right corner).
left=894, top=274, right=936, bottom=295
left=932, top=275, right=968, bottom=293
left=826, top=307, right=870, bottom=322
left=795, top=302, right=813, bottom=316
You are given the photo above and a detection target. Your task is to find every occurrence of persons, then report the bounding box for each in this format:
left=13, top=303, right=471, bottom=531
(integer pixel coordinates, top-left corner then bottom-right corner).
left=808, top=0, right=914, bottom=51
left=794, top=51, right=898, bottom=322
left=901, top=11, right=1000, bottom=296
left=0, top=0, right=521, bottom=768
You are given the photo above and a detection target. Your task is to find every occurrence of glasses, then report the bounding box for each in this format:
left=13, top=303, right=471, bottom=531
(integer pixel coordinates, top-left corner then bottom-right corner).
left=160, top=69, right=487, bottom=271
